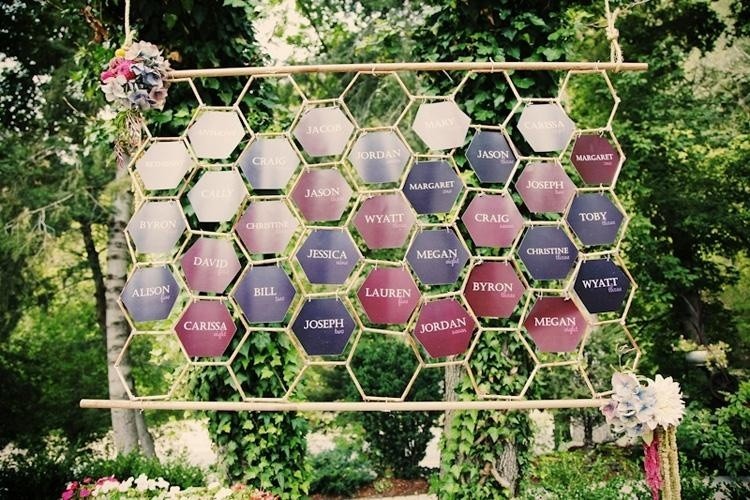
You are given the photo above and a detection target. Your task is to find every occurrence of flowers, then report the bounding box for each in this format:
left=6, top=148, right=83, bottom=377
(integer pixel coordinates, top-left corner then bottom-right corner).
left=599, top=371, right=685, bottom=500
left=101, top=37, right=174, bottom=169
